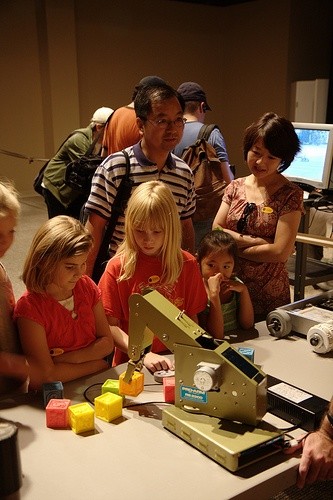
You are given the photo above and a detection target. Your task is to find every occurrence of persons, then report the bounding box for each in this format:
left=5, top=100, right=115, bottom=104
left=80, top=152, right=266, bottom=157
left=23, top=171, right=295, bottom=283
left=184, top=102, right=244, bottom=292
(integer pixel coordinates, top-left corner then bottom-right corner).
left=212, top=112, right=305, bottom=324
left=12, top=215, right=115, bottom=391
left=196, top=230, right=255, bottom=342
left=99, top=181, right=209, bottom=368
left=0, top=180, right=46, bottom=408
left=283, top=393, right=333, bottom=489
left=82, top=83, right=198, bottom=285
left=101, top=75, right=165, bottom=159
left=41, top=106, right=115, bottom=220
left=173, top=81, right=233, bottom=250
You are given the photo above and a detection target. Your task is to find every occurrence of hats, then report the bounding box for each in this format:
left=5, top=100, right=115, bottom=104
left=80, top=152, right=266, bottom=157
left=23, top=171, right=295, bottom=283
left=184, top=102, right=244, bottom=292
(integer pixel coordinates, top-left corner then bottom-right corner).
left=139, top=76, right=168, bottom=87
left=177, top=82, right=212, bottom=112
left=92, top=107, right=115, bottom=125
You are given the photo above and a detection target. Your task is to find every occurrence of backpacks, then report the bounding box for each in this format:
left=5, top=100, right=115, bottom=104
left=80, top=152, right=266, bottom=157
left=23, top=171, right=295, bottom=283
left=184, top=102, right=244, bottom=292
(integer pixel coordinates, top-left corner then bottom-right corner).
left=180, top=123, right=235, bottom=226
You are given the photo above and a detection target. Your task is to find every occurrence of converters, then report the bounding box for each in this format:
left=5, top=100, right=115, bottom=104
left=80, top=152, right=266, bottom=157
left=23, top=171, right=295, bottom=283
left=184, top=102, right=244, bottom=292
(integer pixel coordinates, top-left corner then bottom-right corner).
left=265, top=375, right=330, bottom=433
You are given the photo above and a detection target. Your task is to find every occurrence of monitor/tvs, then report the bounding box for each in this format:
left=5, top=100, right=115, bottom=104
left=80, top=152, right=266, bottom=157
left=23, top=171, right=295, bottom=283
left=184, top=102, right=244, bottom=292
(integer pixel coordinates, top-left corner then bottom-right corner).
left=278, top=121, right=333, bottom=197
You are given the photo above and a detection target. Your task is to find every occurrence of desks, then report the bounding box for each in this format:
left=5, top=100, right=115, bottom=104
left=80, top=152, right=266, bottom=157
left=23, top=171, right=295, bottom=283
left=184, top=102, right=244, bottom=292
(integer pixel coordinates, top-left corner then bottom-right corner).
left=1, top=320, right=333, bottom=500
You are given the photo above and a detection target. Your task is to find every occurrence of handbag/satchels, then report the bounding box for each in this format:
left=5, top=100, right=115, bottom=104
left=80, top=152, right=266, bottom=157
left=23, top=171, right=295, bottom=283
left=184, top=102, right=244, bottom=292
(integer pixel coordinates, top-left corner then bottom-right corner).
left=33, top=159, right=49, bottom=195
left=78, top=149, right=135, bottom=285
left=64, top=155, right=106, bottom=191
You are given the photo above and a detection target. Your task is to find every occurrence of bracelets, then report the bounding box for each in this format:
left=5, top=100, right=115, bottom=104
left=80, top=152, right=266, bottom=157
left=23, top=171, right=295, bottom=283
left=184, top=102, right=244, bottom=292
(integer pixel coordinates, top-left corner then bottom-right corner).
left=325, top=411, right=333, bottom=425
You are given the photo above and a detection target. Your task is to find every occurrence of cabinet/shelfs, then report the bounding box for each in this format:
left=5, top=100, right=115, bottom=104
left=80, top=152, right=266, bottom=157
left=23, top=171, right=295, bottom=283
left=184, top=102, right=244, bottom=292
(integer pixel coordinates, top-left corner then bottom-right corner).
left=286, top=199, right=333, bottom=302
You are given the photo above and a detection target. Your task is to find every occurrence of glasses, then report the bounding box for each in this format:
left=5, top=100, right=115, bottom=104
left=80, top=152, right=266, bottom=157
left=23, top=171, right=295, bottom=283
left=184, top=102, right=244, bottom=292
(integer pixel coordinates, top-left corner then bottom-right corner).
left=237, top=203, right=257, bottom=235
left=139, top=114, right=188, bottom=129
